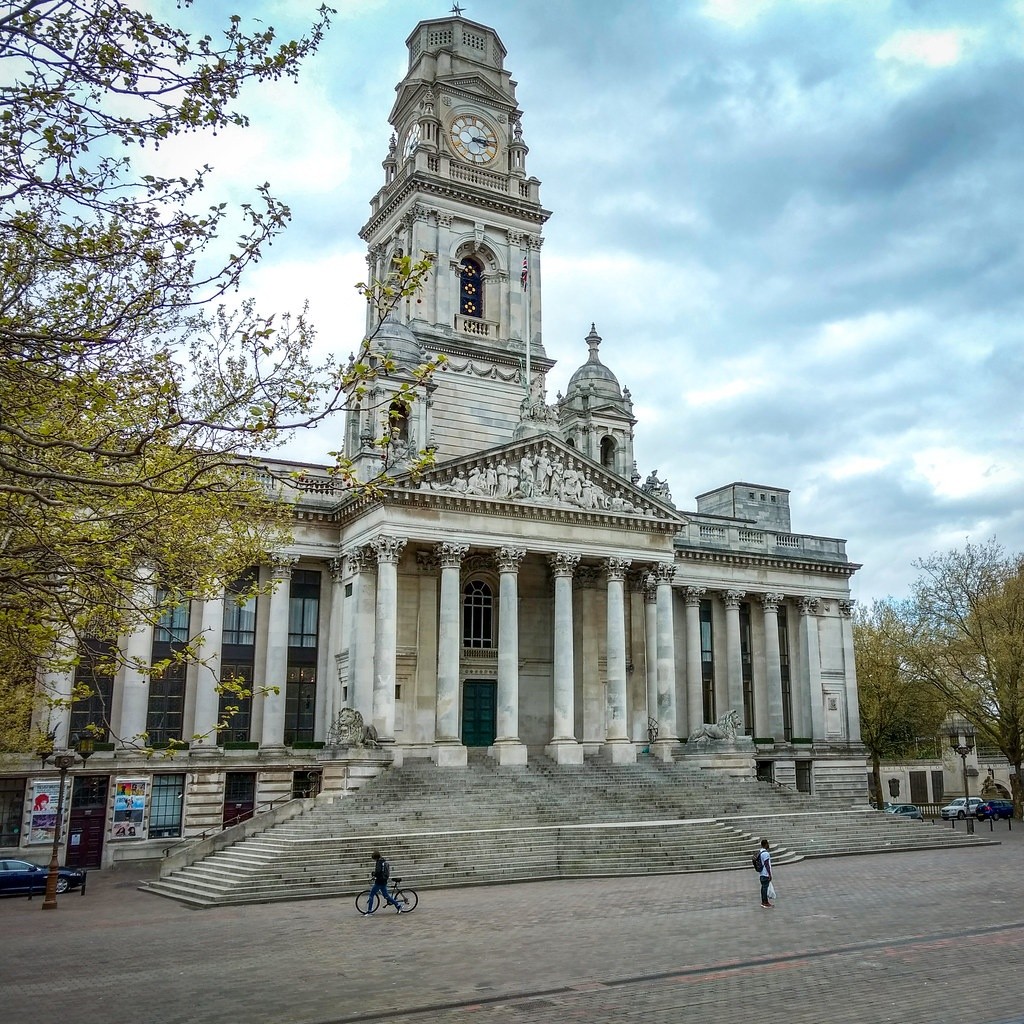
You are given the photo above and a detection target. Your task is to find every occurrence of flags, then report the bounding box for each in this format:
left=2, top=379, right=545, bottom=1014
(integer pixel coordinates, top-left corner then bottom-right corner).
left=520, top=256, right=528, bottom=292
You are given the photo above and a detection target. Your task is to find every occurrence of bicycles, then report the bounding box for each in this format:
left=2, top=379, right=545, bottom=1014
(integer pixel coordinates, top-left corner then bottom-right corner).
left=356, top=876, right=418, bottom=915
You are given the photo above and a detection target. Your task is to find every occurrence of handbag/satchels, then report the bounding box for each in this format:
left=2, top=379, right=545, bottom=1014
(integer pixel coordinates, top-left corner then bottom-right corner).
left=767, top=882, right=776, bottom=899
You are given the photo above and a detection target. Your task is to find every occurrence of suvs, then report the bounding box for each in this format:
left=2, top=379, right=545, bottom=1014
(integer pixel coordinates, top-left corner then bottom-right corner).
left=941, top=797, right=984, bottom=821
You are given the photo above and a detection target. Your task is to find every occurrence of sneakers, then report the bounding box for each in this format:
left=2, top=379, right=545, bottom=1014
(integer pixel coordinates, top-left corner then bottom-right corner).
left=763, top=903, right=774, bottom=908
left=761, top=904, right=762, bottom=906
left=363, top=912, right=373, bottom=917
left=397, top=904, right=404, bottom=915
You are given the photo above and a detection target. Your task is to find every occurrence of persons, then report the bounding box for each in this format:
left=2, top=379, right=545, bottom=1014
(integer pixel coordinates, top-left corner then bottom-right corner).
left=758, top=840, right=774, bottom=908
left=359, top=851, right=404, bottom=917
left=646, top=470, right=668, bottom=489
left=445, top=448, right=633, bottom=512
left=34, top=794, right=49, bottom=811
left=127, top=797, right=133, bottom=808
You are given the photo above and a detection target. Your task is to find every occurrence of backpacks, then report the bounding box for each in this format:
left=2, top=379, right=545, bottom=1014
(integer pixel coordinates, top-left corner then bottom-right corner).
left=380, top=860, right=389, bottom=880
left=752, top=849, right=766, bottom=873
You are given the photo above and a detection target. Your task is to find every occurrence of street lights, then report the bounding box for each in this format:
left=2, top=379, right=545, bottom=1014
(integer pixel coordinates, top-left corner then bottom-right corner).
left=947, top=726, right=975, bottom=835
left=36, top=721, right=95, bottom=910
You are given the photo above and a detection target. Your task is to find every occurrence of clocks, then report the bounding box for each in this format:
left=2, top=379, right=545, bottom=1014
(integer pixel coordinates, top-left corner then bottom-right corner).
left=444, top=112, right=502, bottom=169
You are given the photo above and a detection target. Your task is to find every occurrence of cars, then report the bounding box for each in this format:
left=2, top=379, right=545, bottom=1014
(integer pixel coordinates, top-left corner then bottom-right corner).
left=0, top=859, right=83, bottom=894
left=871, top=802, right=923, bottom=822
left=975, top=800, right=1013, bottom=822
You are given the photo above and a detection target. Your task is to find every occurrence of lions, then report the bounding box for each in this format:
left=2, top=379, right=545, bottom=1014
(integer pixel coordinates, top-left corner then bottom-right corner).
left=334, top=708, right=378, bottom=750
left=687, top=708, right=742, bottom=745
